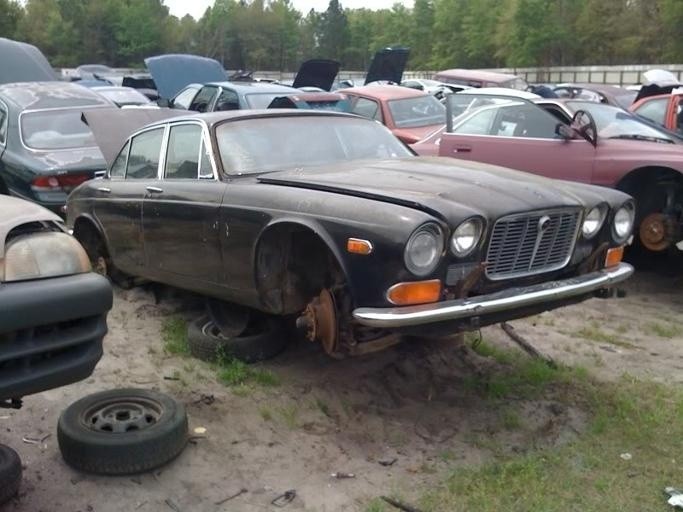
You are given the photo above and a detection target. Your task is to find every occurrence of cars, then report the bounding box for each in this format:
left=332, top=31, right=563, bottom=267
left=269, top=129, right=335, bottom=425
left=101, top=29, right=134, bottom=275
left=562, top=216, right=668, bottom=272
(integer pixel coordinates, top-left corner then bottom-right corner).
left=64, top=109, right=637, bottom=358
